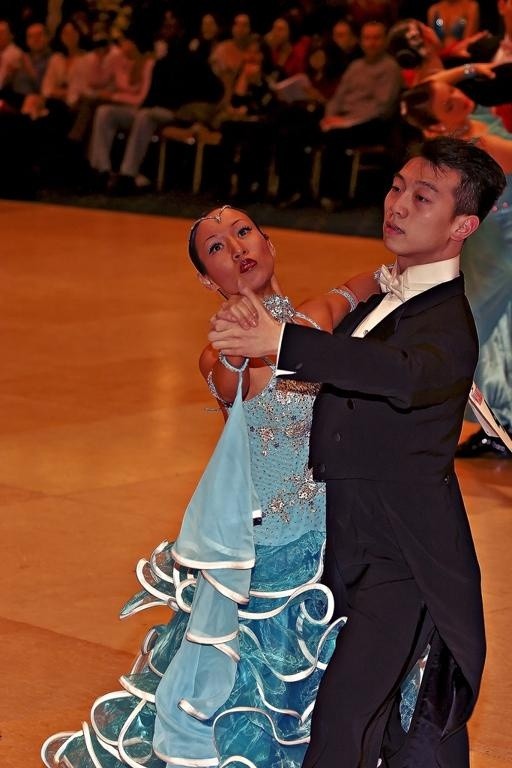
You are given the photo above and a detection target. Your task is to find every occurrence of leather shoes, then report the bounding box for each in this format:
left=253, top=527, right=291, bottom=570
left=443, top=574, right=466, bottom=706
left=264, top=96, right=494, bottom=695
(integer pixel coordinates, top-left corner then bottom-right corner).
left=456, top=423, right=512, bottom=459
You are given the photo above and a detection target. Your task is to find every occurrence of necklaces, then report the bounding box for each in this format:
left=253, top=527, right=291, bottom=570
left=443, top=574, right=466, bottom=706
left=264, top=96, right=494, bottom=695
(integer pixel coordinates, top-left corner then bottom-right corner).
left=259, top=288, right=297, bottom=326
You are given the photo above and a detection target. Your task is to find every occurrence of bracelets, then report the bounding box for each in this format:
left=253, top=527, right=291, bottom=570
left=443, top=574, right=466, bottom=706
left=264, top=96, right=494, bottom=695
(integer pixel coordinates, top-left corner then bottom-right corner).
left=217, top=351, right=251, bottom=374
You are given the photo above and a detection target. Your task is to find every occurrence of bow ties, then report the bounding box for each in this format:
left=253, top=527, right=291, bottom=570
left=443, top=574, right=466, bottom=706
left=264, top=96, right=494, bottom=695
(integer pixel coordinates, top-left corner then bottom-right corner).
left=376, top=264, right=405, bottom=303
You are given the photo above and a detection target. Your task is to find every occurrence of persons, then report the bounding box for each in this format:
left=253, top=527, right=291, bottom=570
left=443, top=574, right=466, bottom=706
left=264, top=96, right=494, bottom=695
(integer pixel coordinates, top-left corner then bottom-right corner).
left=203, top=134, right=512, bottom=766
left=33, top=192, right=413, bottom=767
left=448, top=62, right=512, bottom=458
left=397, top=58, right=512, bottom=458
left=1, top=2, right=512, bottom=205
